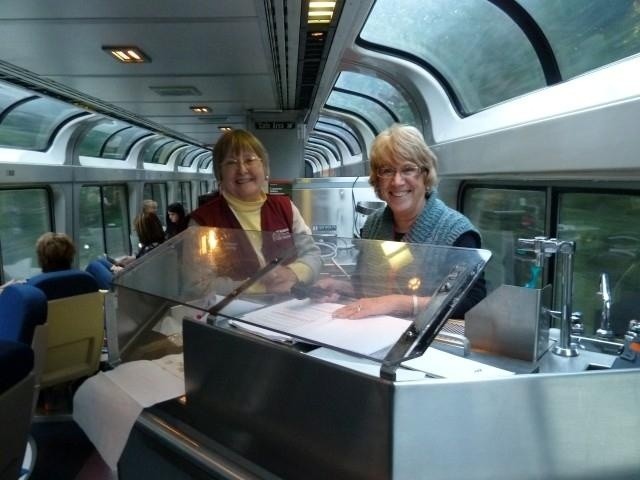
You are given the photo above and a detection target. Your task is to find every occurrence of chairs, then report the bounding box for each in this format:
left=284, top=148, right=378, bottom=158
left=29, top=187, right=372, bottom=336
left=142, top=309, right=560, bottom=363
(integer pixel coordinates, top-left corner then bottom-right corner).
left=0, top=254, right=117, bottom=479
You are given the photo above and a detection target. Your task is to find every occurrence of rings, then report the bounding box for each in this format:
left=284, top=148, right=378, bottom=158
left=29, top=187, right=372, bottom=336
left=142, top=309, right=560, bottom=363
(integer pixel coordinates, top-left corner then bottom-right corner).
left=356, top=305, right=362, bottom=311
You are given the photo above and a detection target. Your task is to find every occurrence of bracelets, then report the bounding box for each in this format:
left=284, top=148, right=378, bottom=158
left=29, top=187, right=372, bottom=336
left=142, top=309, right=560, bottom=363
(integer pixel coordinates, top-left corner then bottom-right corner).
left=411, top=294, right=419, bottom=317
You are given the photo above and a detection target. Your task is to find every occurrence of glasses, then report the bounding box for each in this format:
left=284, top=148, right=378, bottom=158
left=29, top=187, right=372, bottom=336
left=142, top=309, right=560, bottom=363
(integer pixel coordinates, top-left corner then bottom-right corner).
left=375, top=164, right=428, bottom=179
left=218, top=157, right=264, bottom=171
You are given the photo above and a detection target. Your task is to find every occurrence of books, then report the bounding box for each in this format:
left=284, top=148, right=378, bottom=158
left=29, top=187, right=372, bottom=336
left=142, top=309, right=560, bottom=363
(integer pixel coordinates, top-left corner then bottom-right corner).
left=151, top=294, right=516, bottom=381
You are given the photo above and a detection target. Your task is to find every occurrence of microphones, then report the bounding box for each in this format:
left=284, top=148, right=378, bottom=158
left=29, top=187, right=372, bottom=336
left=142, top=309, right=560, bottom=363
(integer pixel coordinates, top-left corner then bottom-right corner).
left=289, top=279, right=354, bottom=302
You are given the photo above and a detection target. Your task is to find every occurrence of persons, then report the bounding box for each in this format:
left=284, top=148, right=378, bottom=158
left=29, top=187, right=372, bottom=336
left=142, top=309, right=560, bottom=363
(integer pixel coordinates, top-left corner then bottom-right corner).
left=307, top=120, right=487, bottom=322
left=183, top=127, right=322, bottom=295
left=0, top=188, right=218, bottom=301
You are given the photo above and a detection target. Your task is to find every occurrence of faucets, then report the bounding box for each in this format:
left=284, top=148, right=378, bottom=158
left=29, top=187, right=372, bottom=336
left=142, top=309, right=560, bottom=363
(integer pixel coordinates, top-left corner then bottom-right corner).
left=596, top=270, right=615, bottom=338
left=532, top=237, right=579, bottom=356
